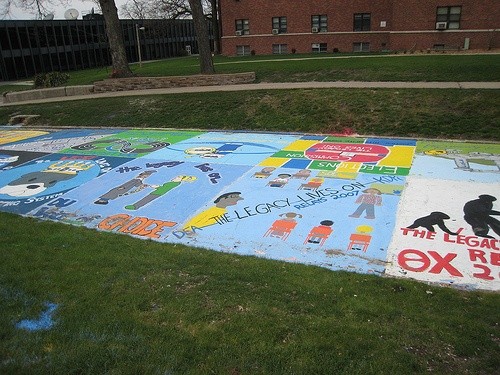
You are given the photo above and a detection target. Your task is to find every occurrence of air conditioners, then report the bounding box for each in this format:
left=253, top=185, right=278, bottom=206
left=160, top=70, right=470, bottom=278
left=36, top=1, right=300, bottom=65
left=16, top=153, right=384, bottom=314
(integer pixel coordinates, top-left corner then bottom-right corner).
left=312, top=43, right=320, bottom=48
left=236, top=30, right=243, bottom=35
left=436, top=22, right=447, bottom=29
left=311, top=26, right=319, bottom=33
left=272, top=28, right=281, bottom=33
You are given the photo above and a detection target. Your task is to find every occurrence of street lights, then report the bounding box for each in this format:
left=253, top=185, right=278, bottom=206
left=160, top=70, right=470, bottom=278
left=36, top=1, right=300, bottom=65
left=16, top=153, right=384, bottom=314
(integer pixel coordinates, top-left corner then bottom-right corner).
left=135, top=24, right=145, bottom=68
